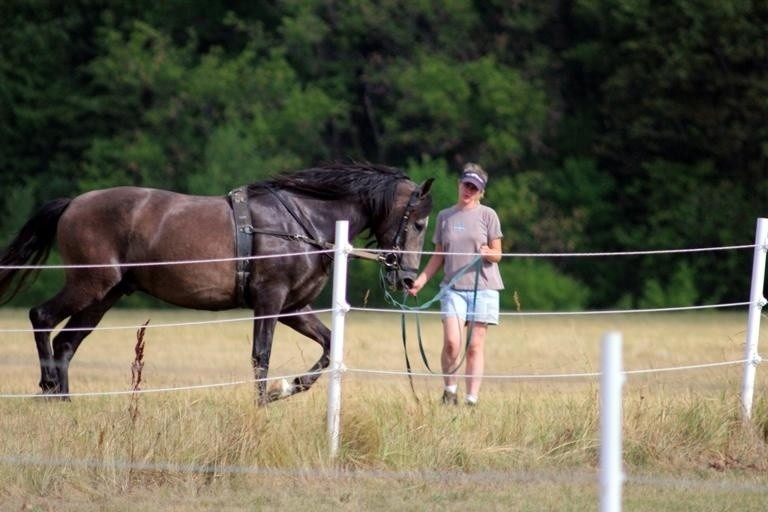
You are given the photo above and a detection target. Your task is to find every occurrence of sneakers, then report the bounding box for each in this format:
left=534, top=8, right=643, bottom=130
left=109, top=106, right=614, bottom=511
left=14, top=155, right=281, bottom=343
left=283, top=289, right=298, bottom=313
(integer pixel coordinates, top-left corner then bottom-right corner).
left=442, top=390, right=457, bottom=405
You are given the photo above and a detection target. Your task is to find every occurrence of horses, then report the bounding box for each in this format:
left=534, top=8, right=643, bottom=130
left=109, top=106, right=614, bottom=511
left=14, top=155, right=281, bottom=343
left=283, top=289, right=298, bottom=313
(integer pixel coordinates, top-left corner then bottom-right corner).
left=0, top=157, right=437, bottom=408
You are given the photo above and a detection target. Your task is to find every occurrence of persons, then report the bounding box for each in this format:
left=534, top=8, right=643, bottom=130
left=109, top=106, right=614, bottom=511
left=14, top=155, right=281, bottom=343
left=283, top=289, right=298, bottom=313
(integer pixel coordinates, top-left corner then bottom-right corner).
left=401, top=162, right=506, bottom=408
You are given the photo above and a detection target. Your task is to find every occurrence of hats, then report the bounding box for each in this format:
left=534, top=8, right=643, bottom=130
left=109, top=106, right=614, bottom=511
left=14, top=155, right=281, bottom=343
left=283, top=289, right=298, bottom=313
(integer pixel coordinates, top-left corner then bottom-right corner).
left=461, top=170, right=486, bottom=191
